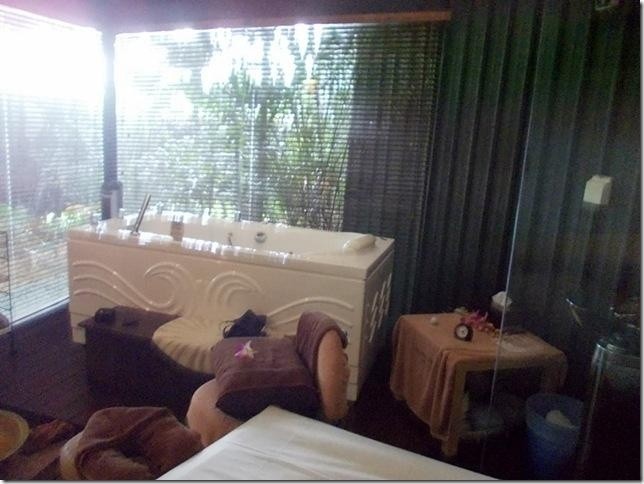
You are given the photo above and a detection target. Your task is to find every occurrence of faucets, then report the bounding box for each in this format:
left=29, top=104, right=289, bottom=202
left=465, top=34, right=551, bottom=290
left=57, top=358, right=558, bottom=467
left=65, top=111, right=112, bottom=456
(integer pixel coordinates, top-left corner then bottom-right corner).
left=130, top=193, right=152, bottom=236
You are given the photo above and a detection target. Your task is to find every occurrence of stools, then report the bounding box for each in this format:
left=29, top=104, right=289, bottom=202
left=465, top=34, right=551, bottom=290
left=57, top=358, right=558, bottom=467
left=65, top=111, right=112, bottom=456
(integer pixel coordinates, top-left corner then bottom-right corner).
left=58, top=431, right=84, bottom=480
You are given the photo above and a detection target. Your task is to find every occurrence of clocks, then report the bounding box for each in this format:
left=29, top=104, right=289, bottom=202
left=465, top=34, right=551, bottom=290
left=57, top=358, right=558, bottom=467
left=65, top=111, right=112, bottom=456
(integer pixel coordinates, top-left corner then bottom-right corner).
left=455, top=323, right=473, bottom=341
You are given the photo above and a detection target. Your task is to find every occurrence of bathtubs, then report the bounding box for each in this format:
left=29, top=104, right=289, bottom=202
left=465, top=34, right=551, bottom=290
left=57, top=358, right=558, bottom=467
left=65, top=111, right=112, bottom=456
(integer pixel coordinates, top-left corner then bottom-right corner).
left=65, top=209, right=396, bottom=403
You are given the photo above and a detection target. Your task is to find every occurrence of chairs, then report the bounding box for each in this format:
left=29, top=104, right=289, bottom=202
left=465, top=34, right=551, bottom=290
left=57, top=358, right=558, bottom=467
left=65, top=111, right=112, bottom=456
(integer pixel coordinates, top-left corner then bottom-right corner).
left=187, top=312, right=351, bottom=450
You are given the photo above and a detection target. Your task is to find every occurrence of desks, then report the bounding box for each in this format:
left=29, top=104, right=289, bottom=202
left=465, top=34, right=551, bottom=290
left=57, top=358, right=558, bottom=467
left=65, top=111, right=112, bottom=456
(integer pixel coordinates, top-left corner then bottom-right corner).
left=388, top=313, right=567, bottom=457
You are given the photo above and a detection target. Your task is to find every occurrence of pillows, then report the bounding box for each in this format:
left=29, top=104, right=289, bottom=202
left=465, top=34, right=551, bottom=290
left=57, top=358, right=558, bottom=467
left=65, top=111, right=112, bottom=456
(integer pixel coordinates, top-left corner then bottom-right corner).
left=210, top=336, right=320, bottom=421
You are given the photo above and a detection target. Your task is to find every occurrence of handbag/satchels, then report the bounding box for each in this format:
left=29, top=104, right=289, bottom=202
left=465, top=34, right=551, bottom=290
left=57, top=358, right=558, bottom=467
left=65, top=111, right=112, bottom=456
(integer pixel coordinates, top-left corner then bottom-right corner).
left=219, top=310, right=267, bottom=336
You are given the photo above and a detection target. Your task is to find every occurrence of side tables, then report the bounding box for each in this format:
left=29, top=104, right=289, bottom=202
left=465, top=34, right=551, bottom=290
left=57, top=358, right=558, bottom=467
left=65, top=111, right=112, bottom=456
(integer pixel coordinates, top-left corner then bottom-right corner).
left=575, top=338, right=641, bottom=471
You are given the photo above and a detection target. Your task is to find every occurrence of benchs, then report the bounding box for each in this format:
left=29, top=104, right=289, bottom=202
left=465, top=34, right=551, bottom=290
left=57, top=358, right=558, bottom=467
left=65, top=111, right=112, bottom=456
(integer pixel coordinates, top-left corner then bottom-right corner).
left=78, top=304, right=215, bottom=410
